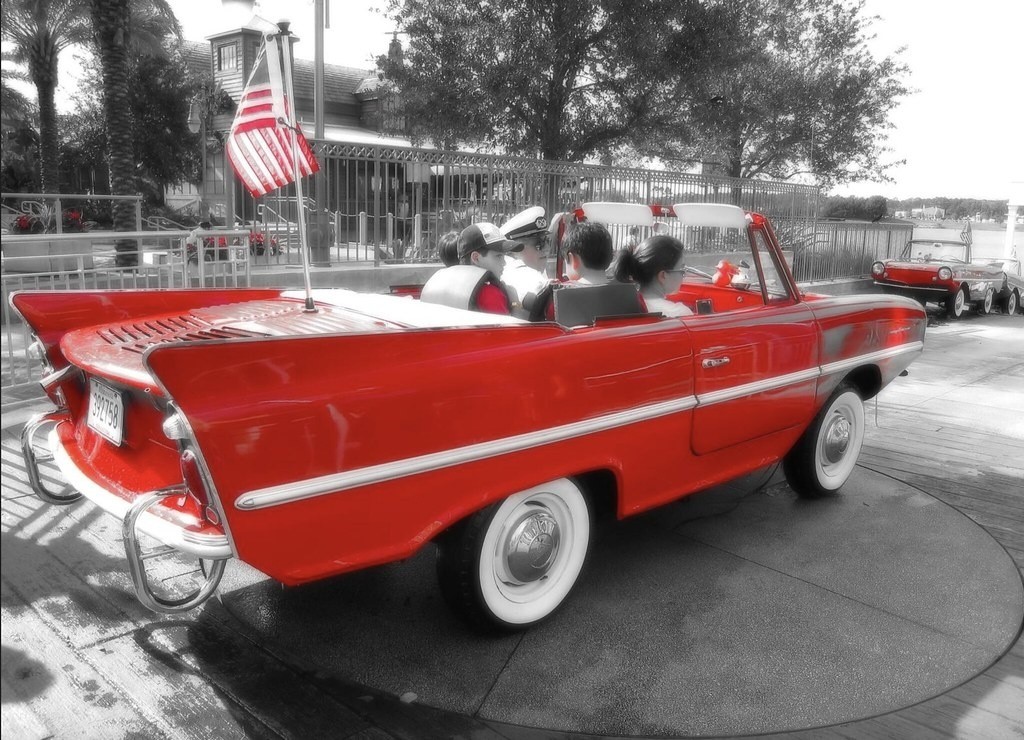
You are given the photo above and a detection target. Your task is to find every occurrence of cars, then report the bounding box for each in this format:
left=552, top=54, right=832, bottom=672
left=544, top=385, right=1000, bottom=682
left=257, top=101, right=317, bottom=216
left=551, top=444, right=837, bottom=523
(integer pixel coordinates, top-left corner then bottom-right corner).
left=869, top=238, right=1024, bottom=320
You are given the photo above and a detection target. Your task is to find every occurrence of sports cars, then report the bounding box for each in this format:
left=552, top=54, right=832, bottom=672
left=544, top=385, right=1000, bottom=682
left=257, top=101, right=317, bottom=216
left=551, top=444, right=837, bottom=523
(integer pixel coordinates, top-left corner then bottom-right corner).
left=8, top=200, right=930, bottom=638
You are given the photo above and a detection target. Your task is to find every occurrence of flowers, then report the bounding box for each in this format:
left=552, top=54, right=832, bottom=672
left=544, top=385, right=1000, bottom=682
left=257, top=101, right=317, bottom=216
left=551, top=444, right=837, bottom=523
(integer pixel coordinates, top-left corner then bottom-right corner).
left=63, top=211, right=93, bottom=231
left=9, top=215, right=46, bottom=235
left=249, top=234, right=285, bottom=256
left=185, top=237, right=227, bottom=258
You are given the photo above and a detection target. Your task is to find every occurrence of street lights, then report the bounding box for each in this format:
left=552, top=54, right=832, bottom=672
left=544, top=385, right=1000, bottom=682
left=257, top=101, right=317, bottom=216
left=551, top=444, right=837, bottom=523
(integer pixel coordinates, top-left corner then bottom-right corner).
left=186, top=80, right=210, bottom=222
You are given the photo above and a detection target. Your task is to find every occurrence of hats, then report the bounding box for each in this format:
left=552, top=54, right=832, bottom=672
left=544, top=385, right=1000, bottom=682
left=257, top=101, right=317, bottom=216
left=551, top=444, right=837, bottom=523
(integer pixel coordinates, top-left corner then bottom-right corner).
left=457, top=222, right=525, bottom=260
left=500, top=206, right=552, bottom=241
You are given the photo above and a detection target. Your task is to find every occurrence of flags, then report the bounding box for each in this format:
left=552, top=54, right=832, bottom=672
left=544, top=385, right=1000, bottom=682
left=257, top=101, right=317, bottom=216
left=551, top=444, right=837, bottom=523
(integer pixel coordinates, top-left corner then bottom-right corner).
left=225, top=33, right=320, bottom=199
left=960, top=220, right=973, bottom=245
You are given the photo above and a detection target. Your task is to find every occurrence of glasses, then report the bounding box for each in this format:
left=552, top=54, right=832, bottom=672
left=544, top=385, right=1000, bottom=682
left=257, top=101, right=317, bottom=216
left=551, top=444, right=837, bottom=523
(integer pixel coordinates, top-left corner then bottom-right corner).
left=534, top=235, right=552, bottom=251
left=666, top=264, right=686, bottom=277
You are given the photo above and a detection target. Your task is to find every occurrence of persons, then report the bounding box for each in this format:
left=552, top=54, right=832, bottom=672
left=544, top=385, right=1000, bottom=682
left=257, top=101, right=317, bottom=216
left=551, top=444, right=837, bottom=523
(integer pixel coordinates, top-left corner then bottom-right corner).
left=186, top=222, right=213, bottom=254
left=616, top=235, right=694, bottom=316
left=419, top=222, right=524, bottom=316
left=497, top=205, right=571, bottom=320
left=546, top=222, right=647, bottom=321
left=438, top=231, right=462, bottom=267
